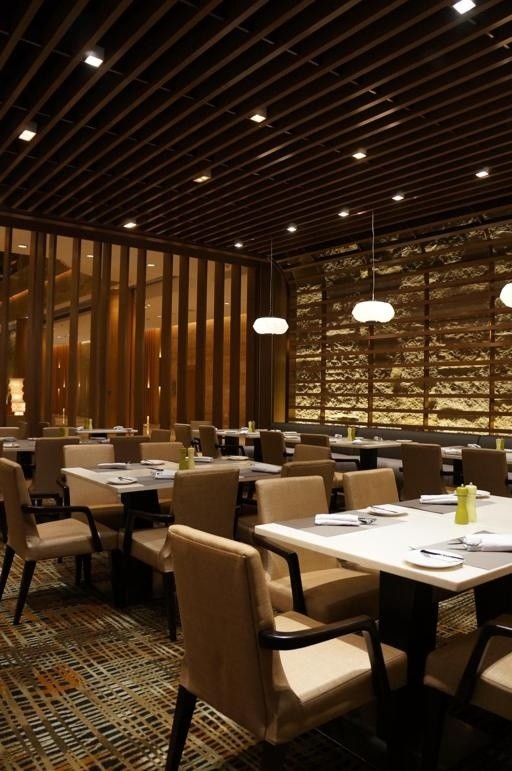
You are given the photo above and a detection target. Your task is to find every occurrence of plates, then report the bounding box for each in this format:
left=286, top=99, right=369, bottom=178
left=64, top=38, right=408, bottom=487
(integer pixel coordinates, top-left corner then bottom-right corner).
left=366, top=503, right=409, bottom=516
left=97, top=461, right=127, bottom=469
left=140, top=459, right=169, bottom=464
left=107, top=476, right=138, bottom=484
left=403, top=548, right=465, bottom=568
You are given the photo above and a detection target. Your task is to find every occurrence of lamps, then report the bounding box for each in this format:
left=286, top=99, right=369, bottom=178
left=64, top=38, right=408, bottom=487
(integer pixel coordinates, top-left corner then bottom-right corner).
left=5, top=375, right=27, bottom=418
left=351, top=208, right=396, bottom=322
left=252, top=227, right=290, bottom=334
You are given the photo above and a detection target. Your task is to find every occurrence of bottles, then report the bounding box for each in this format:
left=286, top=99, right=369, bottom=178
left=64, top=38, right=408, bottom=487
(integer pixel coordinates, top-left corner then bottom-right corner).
left=178, top=445, right=186, bottom=469
left=466, top=481, right=477, bottom=522
left=347, top=425, right=355, bottom=440
left=455, top=482, right=469, bottom=524
left=186, top=444, right=195, bottom=469
left=248, top=419, right=255, bottom=431
left=496, top=435, right=505, bottom=450
